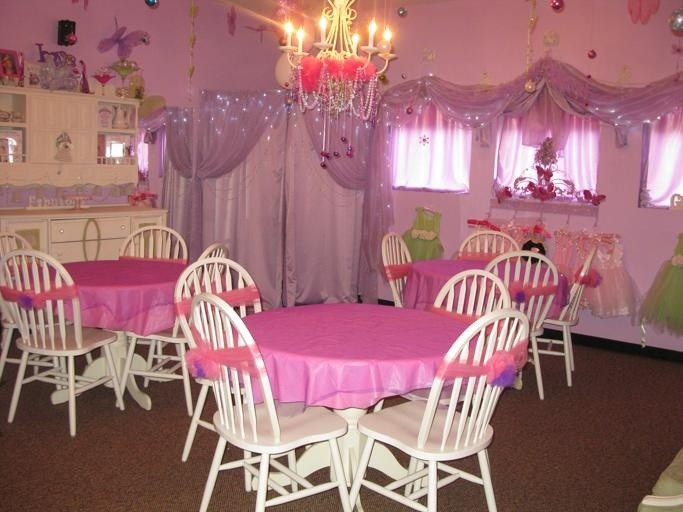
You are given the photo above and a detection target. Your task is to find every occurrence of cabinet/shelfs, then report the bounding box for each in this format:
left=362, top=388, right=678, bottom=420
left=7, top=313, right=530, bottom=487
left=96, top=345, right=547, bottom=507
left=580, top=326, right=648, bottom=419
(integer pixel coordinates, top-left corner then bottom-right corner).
left=1, top=85, right=168, bottom=267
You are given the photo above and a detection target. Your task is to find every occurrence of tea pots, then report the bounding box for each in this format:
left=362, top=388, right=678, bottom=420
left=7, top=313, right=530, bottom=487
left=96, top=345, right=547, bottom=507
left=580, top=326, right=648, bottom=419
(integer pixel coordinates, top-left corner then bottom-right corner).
left=111, top=105, right=130, bottom=129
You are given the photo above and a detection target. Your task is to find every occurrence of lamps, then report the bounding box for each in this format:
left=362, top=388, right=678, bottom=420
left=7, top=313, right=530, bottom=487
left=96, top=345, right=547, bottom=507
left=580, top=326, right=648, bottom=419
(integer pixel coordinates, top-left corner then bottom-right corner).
left=274, top=0, right=398, bottom=168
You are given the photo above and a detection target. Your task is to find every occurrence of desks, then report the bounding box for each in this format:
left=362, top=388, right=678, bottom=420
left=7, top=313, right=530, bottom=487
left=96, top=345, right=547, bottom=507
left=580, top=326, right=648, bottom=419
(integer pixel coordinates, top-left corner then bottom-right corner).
left=231, top=303, right=486, bottom=493
left=10, top=261, right=192, bottom=412
left=403, top=260, right=568, bottom=329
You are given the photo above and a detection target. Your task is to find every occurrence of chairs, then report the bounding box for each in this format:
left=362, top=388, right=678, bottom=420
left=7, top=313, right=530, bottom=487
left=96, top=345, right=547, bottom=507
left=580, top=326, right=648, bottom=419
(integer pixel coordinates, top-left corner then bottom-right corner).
left=349, top=310, right=530, bottom=512
left=0, top=249, right=125, bottom=437
left=1, top=231, right=73, bottom=384
left=191, top=293, right=351, bottom=512
left=381, top=232, right=414, bottom=309
left=482, top=249, right=558, bottom=401
left=116, top=242, right=230, bottom=418
left=455, top=230, right=520, bottom=261
left=173, top=257, right=264, bottom=493
left=525, top=246, right=598, bottom=387
left=370, top=269, right=511, bottom=412
left=117, top=225, right=188, bottom=387
